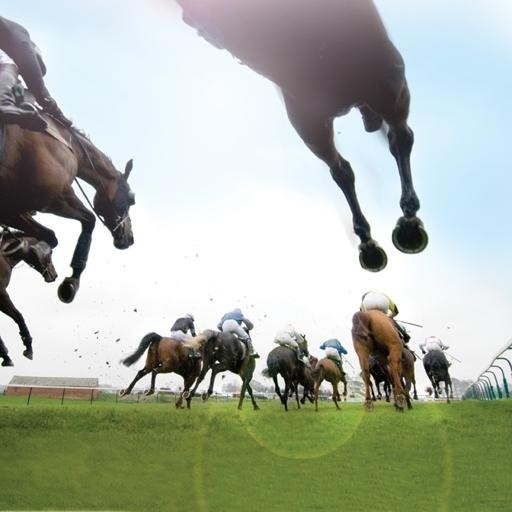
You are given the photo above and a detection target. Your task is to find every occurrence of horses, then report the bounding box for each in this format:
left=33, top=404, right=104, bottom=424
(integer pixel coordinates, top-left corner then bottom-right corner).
left=0, top=232, right=58, bottom=366
left=267, top=335, right=347, bottom=411
left=182, top=327, right=260, bottom=410
left=123, top=325, right=220, bottom=408
left=352, top=309, right=453, bottom=411
left=178, top=0, right=429, bottom=272
left=1, top=111, right=135, bottom=304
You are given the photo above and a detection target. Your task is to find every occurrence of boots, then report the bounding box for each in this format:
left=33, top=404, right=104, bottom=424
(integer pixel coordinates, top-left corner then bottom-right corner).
left=188, top=349, right=200, bottom=360
left=1, top=63, right=37, bottom=124
left=246, top=340, right=259, bottom=359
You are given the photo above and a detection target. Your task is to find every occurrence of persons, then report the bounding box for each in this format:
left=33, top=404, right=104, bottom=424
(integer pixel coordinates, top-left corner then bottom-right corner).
left=216, top=306, right=261, bottom=358
left=275, top=330, right=307, bottom=364
left=359, top=287, right=412, bottom=344
left=0, top=14, right=80, bottom=135
left=317, top=336, right=348, bottom=375
left=168, top=313, right=203, bottom=360
left=416, top=334, right=452, bottom=368
